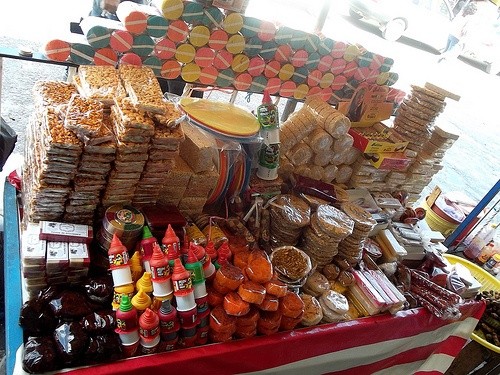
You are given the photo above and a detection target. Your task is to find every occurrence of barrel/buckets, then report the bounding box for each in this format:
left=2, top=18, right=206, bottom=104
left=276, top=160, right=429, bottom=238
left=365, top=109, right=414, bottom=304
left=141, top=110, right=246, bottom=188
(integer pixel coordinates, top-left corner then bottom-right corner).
left=415, top=190, right=486, bottom=247
left=95, top=202, right=145, bottom=262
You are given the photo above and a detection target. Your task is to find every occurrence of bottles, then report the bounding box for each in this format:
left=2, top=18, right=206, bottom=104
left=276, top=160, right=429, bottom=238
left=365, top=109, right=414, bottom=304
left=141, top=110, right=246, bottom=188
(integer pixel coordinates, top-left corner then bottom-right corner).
left=462, top=222, right=500, bottom=271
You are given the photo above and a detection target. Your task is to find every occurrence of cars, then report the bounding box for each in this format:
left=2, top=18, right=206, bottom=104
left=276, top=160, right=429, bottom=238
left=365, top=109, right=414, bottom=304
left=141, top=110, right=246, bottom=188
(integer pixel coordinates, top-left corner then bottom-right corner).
left=454, top=0, right=500, bottom=75
left=348, top=0, right=465, bottom=59
